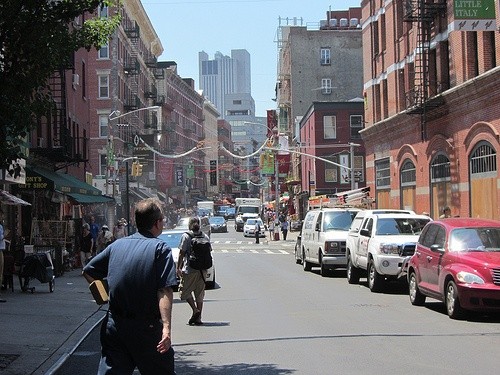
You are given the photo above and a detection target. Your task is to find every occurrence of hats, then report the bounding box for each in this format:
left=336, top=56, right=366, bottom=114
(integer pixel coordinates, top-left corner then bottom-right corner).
left=117, top=221, right=124, bottom=226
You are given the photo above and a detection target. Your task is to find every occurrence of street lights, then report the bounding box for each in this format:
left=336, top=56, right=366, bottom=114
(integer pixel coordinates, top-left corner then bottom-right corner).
left=182, top=146, right=211, bottom=210
left=106, top=105, right=159, bottom=196
left=243, top=122, right=279, bottom=241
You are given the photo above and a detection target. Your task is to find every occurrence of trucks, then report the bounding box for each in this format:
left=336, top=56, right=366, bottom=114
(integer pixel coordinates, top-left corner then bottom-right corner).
left=197, top=202, right=214, bottom=218
left=234, top=198, right=261, bottom=232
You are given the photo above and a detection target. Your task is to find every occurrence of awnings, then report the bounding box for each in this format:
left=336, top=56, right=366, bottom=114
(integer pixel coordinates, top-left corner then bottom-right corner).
left=69, top=193, right=114, bottom=204
left=193, top=196, right=203, bottom=201
left=10, top=165, right=102, bottom=196
left=128, top=188, right=182, bottom=204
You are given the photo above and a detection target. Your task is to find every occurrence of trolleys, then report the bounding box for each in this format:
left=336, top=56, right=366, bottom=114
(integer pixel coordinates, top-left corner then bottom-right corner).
left=18, top=243, right=56, bottom=293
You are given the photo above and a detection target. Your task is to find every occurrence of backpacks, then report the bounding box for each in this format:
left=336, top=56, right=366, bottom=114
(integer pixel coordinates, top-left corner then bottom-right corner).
left=184, top=231, right=212, bottom=271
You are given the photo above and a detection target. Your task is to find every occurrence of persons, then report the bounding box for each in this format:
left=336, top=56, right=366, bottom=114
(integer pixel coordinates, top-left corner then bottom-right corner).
left=162, top=208, right=207, bottom=229
left=253, top=207, right=289, bottom=244
left=177, top=216, right=212, bottom=325
left=440, top=207, right=453, bottom=219
left=77, top=214, right=128, bottom=269
left=0, top=209, right=11, bottom=302
left=81, top=200, right=178, bottom=375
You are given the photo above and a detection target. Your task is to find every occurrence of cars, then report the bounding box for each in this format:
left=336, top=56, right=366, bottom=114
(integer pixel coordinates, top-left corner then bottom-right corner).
left=172, top=215, right=212, bottom=239
left=224, top=208, right=236, bottom=220
left=406, top=218, right=500, bottom=320
left=294, top=234, right=302, bottom=264
left=243, top=219, right=266, bottom=238
left=208, top=217, right=228, bottom=233
left=157, top=229, right=215, bottom=289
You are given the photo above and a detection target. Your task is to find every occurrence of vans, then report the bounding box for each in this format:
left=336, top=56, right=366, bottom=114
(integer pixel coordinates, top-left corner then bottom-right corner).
left=300, top=207, right=363, bottom=277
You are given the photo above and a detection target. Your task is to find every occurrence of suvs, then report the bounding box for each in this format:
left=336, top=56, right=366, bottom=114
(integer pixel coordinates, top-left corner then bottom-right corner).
left=217, top=206, right=230, bottom=216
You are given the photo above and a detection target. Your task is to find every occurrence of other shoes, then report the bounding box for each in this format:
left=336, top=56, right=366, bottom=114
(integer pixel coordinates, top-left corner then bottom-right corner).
left=188, top=309, right=203, bottom=326
left=0, top=296, right=7, bottom=302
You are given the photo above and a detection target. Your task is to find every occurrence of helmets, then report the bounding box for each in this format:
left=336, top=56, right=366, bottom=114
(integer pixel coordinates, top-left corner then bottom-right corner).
left=101, top=225, right=109, bottom=230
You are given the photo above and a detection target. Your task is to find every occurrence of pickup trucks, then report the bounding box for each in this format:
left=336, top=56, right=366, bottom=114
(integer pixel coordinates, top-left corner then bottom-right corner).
left=346, top=209, right=433, bottom=293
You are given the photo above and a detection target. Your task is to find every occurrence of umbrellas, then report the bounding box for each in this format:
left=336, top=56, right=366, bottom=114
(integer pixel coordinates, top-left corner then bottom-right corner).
left=0, top=188, right=31, bottom=205
